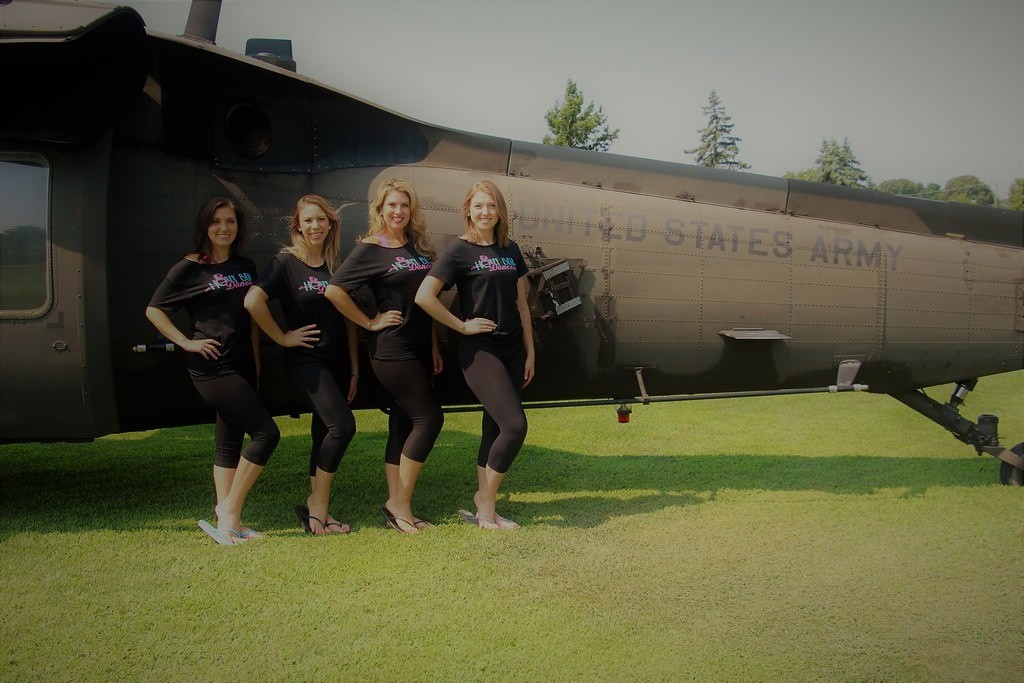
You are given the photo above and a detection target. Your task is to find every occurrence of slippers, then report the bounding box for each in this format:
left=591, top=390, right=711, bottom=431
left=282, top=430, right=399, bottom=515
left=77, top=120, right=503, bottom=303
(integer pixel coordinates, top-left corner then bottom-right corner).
left=295, top=505, right=327, bottom=536
left=458, top=510, right=496, bottom=526
left=198, top=520, right=242, bottom=545
left=379, top=507, right=413, bottom=533
left=241, top=530, right=256, bottom=538
left=326, top=521, right=351, bottom=533
left=414, top=520, right=428, bottom=529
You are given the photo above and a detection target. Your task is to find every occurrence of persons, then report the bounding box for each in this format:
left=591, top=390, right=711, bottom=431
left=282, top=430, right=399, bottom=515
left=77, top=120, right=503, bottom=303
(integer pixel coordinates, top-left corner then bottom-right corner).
left=145, top=178, right=536, bottom=545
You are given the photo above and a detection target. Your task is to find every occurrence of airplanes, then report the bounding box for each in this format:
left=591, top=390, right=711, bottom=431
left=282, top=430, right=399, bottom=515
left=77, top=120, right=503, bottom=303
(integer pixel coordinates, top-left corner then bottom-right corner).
left=1, top=3, right=1023, bottom=488
